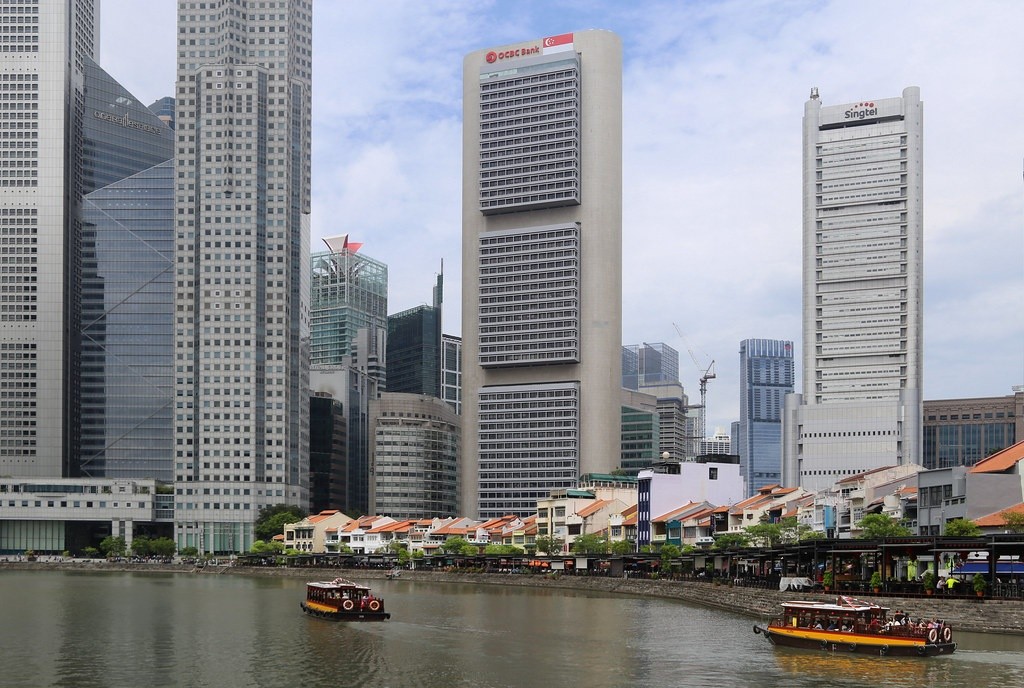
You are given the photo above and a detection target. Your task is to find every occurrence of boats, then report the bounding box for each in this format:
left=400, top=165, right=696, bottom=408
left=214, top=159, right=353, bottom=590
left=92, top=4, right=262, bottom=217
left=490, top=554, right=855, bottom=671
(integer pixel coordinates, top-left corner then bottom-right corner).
left=752, top=600, right=958, bottom=656
left=299, top=579, right=391, bottom=621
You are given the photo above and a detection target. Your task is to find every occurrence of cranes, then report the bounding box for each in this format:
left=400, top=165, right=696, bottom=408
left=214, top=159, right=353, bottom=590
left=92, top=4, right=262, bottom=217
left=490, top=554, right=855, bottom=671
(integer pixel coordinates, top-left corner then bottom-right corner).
left=672, top=322, right=717, bottom=405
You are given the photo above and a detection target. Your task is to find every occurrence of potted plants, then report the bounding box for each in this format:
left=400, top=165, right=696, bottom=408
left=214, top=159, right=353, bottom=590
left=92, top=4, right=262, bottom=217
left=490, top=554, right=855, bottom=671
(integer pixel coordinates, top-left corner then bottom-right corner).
left=924, top=572, right=935, bottom=594
left=823, top=574, right=832, bottom=591
left=972, top=575, right=985, bottom=597
left=871, top=572, right=880, bottom=592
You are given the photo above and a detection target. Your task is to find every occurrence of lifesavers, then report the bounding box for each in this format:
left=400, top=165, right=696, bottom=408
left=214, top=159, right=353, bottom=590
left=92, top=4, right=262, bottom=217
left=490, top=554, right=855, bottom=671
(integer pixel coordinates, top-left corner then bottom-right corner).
left=849, top=642, right=857, bottom=651
left=916, top=645, right=926, bottom=655
left=369, top=600, right=380, bottom=611
left=344, top=600, right=354, bottom=611
left=881, top=644, right=889, bottom=655
left=819, top=638, right=828, bottom=648
left=300, top=601, right=335, bottom=619
left=929, top=629, right=938, bottom=642
left=943, top=628, right=951, bottom=641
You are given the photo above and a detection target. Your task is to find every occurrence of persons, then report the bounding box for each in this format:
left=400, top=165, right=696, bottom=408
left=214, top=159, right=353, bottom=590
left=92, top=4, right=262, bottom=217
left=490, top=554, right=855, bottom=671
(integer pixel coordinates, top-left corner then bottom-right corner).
left=406, top=559, right=851, bottom=595
left=808, top=607, right=945, bottom=642
left=18, top=550, right=376, bottom=611
left=887, top=570, right=1024, bottom=598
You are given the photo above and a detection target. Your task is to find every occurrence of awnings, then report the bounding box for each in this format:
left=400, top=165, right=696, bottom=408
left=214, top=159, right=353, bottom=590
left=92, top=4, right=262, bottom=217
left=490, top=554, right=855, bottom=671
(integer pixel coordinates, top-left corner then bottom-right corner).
left=948, top=563, right=1024, bottom=574
